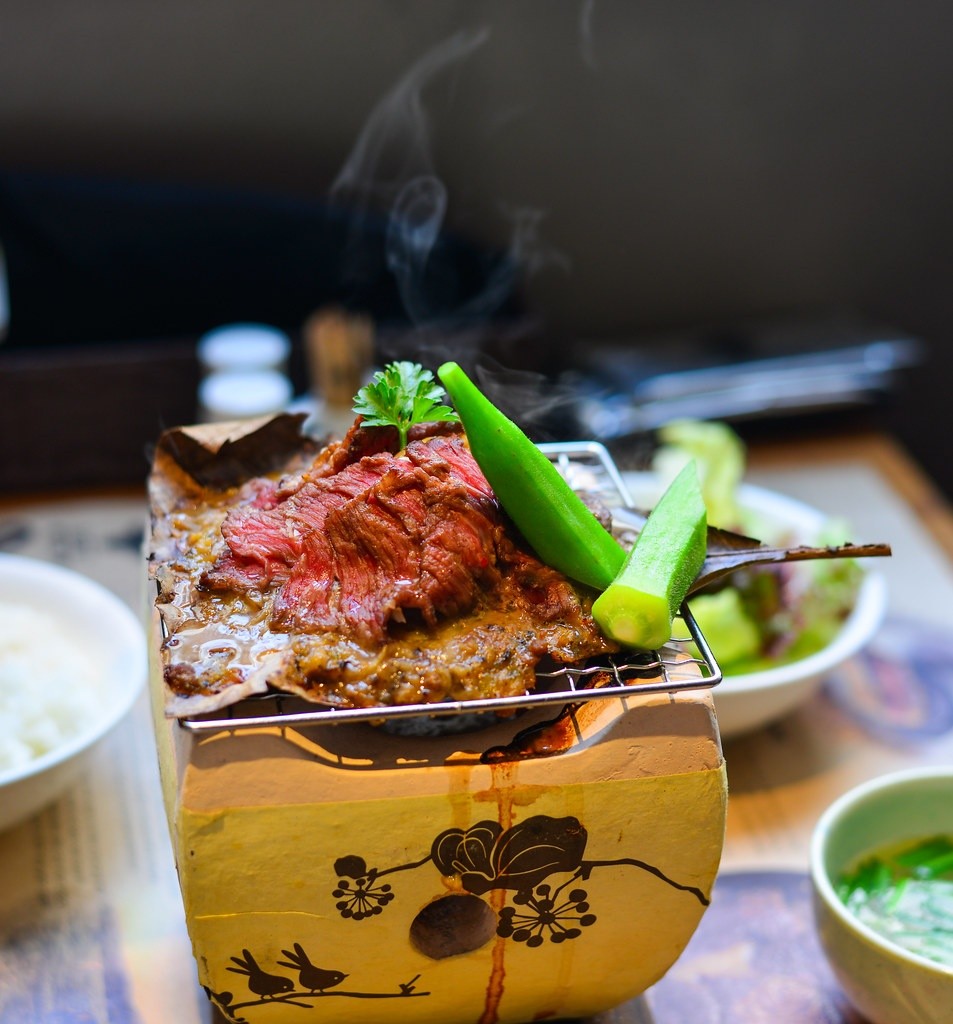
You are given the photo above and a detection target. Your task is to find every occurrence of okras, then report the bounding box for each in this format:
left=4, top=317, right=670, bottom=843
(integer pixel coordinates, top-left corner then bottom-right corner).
left=435, top=349, right=709, bottom=651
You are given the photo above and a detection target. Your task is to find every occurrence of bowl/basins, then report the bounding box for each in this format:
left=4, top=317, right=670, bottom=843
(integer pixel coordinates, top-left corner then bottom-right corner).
left=810, top=765, right=953, bottom=1024
left=0, top=553, right=148, bottom=833
left=615, top=471, right=885, bottom=739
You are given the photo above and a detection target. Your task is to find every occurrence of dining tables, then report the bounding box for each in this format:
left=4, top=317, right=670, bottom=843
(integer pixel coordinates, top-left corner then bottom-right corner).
left=1, top=418, right=952, bottom=1022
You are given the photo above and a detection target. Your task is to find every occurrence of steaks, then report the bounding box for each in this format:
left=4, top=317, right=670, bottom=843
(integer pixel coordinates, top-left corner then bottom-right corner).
left=193, top=395, right=610, bottom=696
